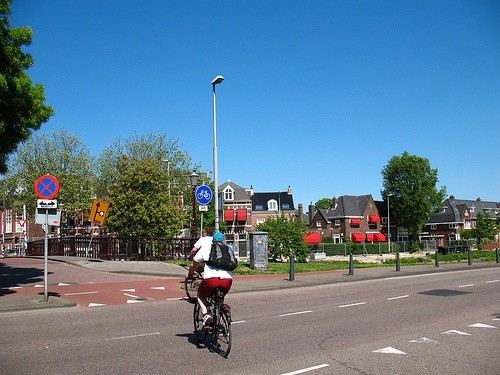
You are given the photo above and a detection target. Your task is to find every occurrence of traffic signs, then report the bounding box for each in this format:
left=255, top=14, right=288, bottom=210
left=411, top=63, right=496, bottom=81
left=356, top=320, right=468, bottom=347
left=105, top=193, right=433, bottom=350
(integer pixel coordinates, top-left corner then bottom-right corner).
left=36, top=198, right=58, bottom=209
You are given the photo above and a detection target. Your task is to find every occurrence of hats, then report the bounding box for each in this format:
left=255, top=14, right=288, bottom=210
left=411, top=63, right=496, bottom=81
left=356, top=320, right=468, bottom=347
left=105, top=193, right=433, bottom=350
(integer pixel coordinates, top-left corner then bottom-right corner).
left=210, top=231, right=225, bottom=242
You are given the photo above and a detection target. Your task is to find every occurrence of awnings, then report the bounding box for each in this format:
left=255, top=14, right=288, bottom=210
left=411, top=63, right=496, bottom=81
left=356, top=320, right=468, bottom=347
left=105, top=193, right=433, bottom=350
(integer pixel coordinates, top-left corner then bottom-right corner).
left=350, top=219, right=362, bottom=224
left=303, top=232, right=320, bottom=244
left=370, top=215, right=380, bottom=222
left=351, top=232, right=386, bottom=242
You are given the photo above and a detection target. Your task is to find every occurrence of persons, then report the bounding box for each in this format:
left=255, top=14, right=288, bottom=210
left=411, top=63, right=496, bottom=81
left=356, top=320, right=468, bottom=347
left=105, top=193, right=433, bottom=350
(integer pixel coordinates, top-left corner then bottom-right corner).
left=185, top=227, right=214, bottom=258
left=186, top=230, right=234, bottom=337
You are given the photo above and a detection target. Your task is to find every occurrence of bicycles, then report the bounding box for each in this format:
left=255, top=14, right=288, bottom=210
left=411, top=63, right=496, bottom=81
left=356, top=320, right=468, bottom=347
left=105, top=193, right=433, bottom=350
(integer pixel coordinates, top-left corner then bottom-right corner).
left=184, top=255, right=202, bottom=301
left=187, top=268, right=233, bottom=357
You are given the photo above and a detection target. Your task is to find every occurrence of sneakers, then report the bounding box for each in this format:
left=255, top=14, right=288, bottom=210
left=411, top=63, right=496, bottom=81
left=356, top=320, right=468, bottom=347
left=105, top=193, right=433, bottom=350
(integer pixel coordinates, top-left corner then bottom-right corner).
left=199, top=313, right=212, bottom=332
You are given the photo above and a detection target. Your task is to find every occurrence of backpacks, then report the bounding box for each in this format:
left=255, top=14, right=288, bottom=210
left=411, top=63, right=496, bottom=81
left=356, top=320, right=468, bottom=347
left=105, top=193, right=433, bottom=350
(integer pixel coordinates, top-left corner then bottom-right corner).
left=202, top=242, right=239, bottom=272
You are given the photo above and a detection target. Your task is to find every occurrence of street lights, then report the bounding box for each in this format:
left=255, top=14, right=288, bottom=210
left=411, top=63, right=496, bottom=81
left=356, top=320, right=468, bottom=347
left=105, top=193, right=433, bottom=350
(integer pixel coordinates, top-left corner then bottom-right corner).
left=387, top=192, right=395, bottom=254
left=212, top=76, right=224, bottom=234
